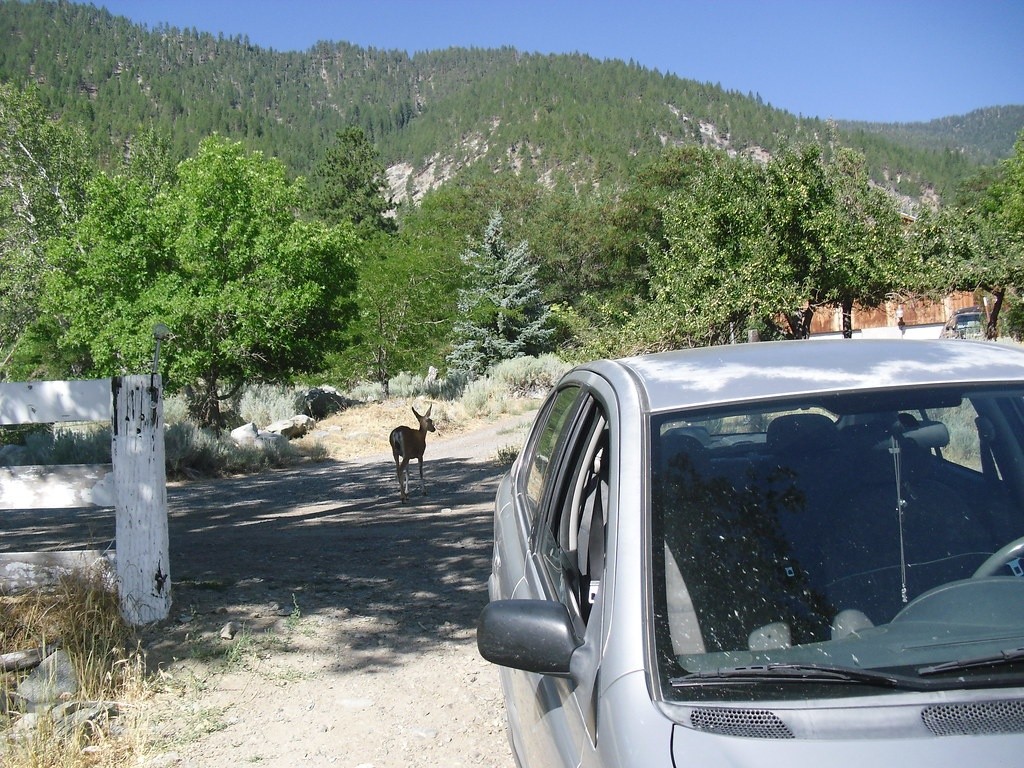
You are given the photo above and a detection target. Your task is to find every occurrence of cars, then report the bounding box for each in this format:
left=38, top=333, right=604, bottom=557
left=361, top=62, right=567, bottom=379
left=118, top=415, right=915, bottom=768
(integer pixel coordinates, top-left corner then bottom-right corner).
left=476, top=340, right=1024, bottom=768
left=940, top=308, right=982, bottom=340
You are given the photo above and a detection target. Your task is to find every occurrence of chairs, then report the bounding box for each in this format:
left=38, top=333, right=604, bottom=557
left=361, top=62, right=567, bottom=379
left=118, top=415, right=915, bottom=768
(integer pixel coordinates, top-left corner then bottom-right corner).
left=863, top=476, right=983, bottom=611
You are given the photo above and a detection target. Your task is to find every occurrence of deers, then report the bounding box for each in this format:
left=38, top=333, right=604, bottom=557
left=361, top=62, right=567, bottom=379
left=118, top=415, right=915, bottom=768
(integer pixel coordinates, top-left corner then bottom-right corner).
left=389, top=403, right=436, bottom=504
